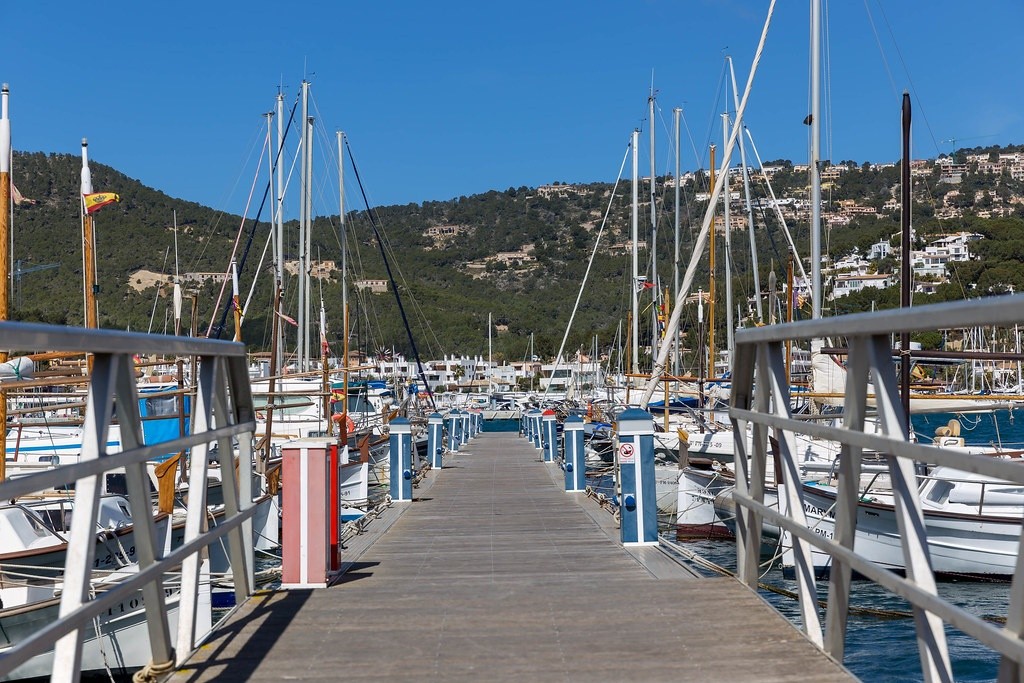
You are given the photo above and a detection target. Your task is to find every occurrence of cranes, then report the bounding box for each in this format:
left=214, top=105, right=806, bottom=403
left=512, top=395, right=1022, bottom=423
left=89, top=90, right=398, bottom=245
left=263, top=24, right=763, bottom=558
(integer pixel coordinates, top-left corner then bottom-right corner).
left=941, top=134, right=1001, bottom=158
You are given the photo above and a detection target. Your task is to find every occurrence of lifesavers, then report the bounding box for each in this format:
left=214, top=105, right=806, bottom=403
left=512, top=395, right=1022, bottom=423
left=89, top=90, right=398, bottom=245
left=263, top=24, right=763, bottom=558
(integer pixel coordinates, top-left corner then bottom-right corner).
left=331, top=414, right=355, bottom=433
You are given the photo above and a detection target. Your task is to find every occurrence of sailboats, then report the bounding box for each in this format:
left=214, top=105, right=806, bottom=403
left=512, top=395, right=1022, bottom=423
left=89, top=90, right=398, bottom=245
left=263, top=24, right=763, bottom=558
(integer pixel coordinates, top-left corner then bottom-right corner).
left=540, top=0, right=1024, bottom=583
left=0, top=54, right=428, bottom=683
